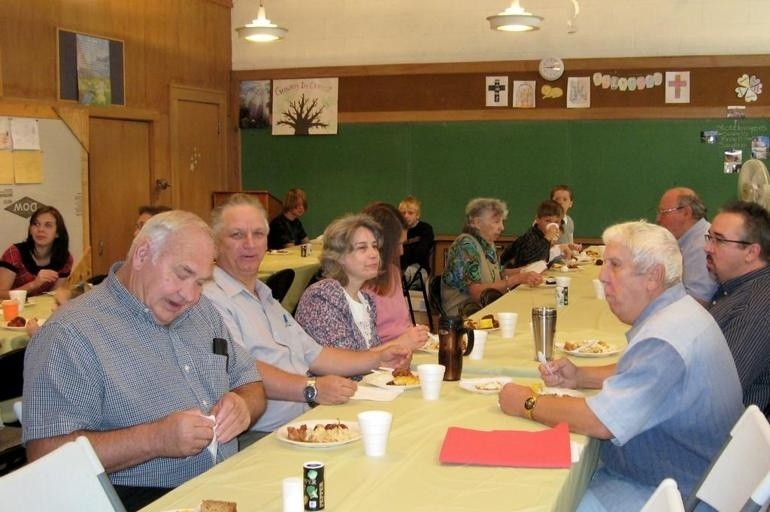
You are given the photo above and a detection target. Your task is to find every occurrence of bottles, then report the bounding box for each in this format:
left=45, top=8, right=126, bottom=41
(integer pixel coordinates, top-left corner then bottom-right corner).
left=301, top=246, right=306, bottom=257
left=532, top=307, right=557, bottom=359
left=438, top=315, right=473, bottom=381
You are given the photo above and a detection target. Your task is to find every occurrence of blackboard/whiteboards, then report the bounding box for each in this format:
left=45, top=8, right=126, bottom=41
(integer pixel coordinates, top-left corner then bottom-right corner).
left=239, top=108, right=769, bottom=242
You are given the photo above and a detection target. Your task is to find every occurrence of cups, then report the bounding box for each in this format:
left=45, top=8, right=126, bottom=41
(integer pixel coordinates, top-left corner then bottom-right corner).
left=303, top=244, right=312, bottom=256
left=357, top=411, right=392, bottom=456
left=498, top=311, right=519, bottom=338
left=592, top=279, right=607, bottom=300
left=546, top=223, right=559, bottom=240
left=467, top=329, right=487, bottom=359
left=557, top=277, right=570, bottom=307
left=2, top=301, right=17, bottom=320
left=8, top=290, right=27, bottom=312
left=417, top=364, right=445, bottom=401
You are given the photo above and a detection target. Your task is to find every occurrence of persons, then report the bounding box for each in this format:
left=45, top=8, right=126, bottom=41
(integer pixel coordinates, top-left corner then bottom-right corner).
left=656, top=187, right=721, bottom=310
left=22, top=210, right=267, bottom=511
left=500, top=218, right=748, bottom=511
left=200, top=202, right=415, bottom=451
left=298, top=193, right=434, bottom=383
left=0, top=206, right=72, bottom=301
left=440, top=187, right=581, bottom=322
left=16, top=288, right=84, bottom=423
left=127, top=206, right=175, bottom=260
left=704, top=200, right=770, bottom=423
left=267, top=190, right=308, bottom=247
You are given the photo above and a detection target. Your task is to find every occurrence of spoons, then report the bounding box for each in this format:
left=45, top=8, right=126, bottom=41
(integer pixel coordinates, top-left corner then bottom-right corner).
left=537, top=350, right=552, bottom=374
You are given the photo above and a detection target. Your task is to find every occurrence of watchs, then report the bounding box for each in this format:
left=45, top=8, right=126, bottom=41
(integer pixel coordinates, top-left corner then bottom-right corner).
left=304, top=377, right=319, bottom=405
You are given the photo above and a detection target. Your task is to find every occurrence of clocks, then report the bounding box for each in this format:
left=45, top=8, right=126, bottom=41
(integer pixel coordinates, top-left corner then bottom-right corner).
left=539, top=56, right=564, bottom=81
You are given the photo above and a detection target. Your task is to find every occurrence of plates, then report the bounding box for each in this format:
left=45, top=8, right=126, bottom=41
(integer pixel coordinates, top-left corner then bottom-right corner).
left=276, top=419, right=360, bottom=448
left=363, top=371, right=420, bottom=389
left=416, top=349, right=466, bottom=356
left=3, top=319, right=47, bottom=331
left=458, top=377, right=511, bottom=394
left=536, top=387, right=584, bottom=399
left=558, top=341, right=621, bottom=356
left=267, top=249, right=289, bottom=254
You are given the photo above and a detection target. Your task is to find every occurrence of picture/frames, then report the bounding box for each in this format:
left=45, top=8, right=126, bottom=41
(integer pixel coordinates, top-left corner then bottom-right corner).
left=57, top=28, right=124, bottom=105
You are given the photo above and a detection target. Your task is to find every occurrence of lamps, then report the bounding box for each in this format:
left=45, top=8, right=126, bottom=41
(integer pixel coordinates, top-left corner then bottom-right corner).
left=235, top=1, right=289, bottom=43
left=487, top=0, right=543, bottom=31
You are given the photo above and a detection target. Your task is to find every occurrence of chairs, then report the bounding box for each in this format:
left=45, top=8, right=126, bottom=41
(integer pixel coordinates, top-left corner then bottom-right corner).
left=403, top=240, right=435, bottom=332
left=681, top=403, right=770, bottom=512
left=266, top=269, right=295, bottom=303
left=429, top=275, right=464, bottom=325
left=2, top=437, right=127, bottom=512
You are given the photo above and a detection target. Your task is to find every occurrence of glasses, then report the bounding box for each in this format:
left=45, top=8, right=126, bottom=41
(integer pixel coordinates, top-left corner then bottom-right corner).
left=658, top=207, right=684, bottom=215
left=704, top=235, right=751, bottom=244
